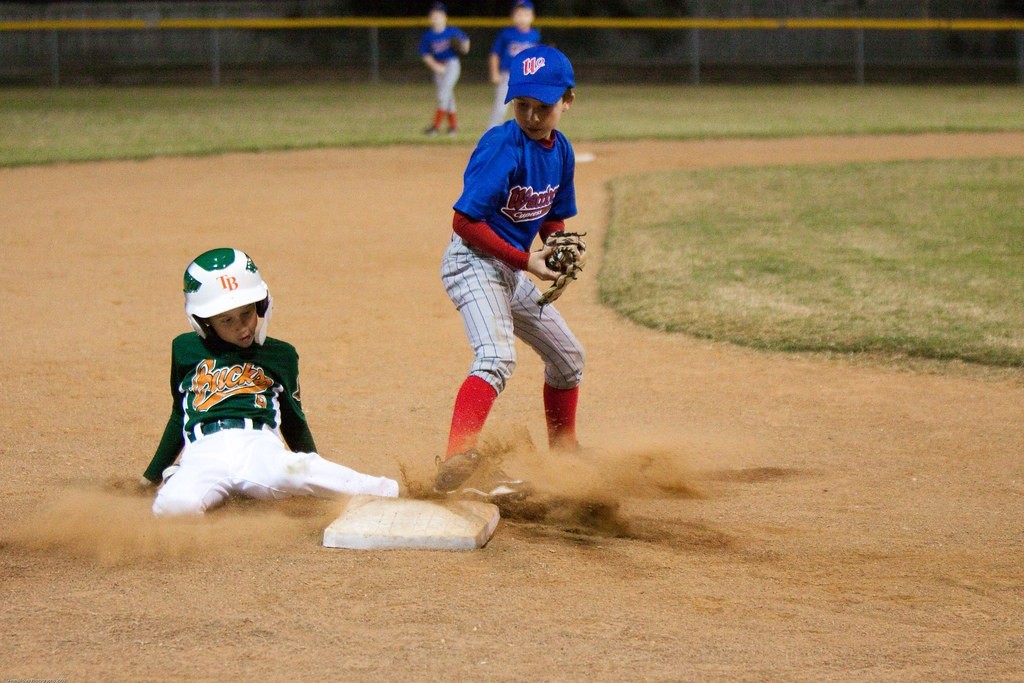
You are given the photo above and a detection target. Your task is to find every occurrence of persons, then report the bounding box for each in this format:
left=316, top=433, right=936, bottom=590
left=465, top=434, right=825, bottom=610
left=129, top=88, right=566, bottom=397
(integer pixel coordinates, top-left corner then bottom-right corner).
left=489, top=0, right=542, bottom=126
left=441, top=46, right=586, bottom=462
left=140, top=248, right=410, bottom=513
left=420, top=7, right=470, bottom=134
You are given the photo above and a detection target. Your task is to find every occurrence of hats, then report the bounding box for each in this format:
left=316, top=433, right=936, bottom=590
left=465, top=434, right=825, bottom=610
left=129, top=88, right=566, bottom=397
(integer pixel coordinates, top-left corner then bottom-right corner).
left=503, top=44, right=575, bottom=105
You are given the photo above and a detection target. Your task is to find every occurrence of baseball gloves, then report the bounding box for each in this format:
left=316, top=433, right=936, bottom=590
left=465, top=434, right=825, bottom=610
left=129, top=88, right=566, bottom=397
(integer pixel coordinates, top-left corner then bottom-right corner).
left=449, top=35, right=461, bottom=53
left=535, top=230, right=587, bottom=309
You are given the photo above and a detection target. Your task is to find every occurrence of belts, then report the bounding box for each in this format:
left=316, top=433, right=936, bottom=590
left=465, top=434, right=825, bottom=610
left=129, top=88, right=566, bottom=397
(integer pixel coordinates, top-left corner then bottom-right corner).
left=188, top=419, right=262, bottom=443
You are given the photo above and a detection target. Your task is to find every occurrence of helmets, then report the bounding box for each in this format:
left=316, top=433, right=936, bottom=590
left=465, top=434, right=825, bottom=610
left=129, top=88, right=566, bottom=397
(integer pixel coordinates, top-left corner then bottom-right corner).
left=182, top=247, right=273, bottom=346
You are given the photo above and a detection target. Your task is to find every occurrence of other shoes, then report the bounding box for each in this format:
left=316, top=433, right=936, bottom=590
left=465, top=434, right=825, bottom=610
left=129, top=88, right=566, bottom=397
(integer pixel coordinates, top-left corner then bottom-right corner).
left=433, top=447, right=529, bottom=500
